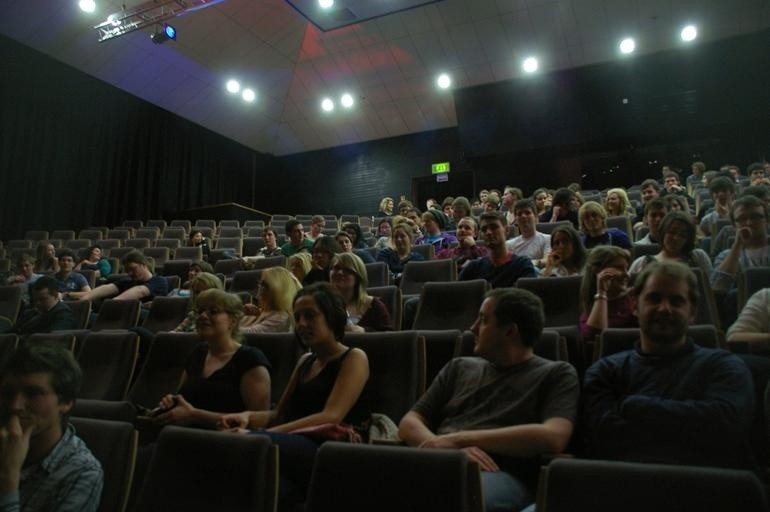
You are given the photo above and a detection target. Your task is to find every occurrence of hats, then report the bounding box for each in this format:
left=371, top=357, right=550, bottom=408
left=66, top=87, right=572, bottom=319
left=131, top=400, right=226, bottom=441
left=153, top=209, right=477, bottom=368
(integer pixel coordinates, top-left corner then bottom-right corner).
left=428, top=210, right=450, bottom=228
left=120, top=251, right=144, bottom=265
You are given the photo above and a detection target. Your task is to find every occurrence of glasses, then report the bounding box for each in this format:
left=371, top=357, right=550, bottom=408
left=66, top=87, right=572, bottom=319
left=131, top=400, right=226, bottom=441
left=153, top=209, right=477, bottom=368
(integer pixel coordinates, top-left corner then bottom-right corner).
left=734, top=212, right=765, bottom=223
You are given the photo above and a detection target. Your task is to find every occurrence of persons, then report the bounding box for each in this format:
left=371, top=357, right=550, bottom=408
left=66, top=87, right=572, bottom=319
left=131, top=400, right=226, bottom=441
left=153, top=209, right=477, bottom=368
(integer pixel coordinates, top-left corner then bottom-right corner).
left=1, top=161, right=769, bottom=372
left=520, top=260, right=756, bottom=511
left=137, top=287, right=274, bottom=433
left=396, top=286, right=581, bottom=510
left=213, top=279, right=374, bottom=444
left=1, top=337, right=105, bottom=512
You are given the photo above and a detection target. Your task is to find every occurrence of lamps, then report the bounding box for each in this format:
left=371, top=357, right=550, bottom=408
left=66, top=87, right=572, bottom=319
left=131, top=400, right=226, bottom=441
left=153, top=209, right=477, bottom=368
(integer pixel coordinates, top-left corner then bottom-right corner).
left=149, top=21, right=176, bottom=45
